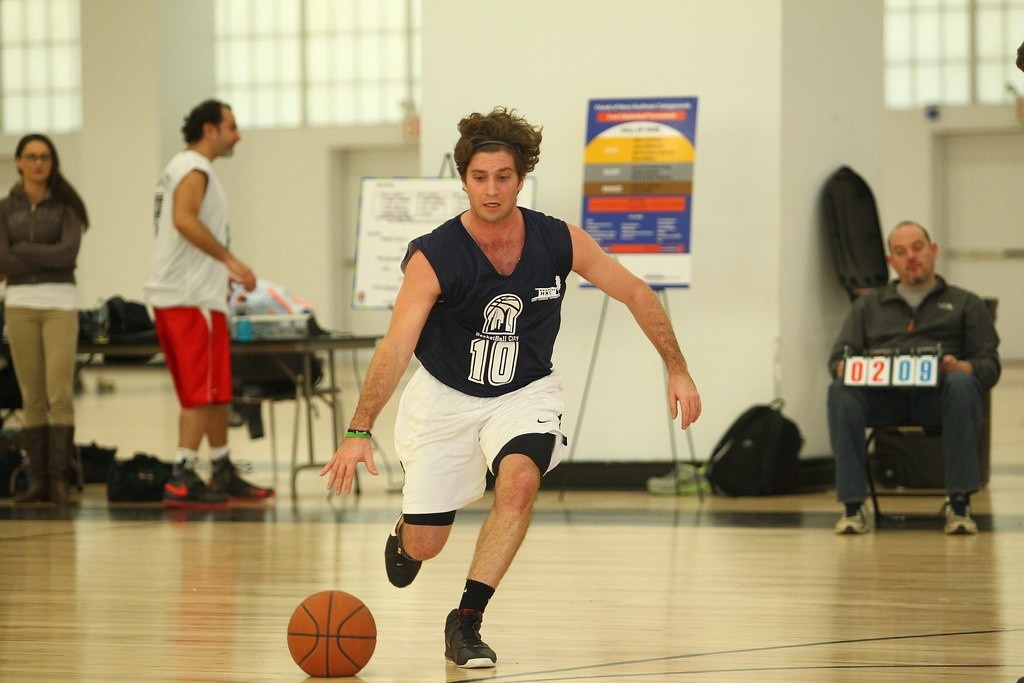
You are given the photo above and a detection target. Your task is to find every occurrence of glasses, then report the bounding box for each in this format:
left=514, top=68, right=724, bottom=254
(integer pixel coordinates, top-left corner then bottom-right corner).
left=24, top=153, right=51, bottom=160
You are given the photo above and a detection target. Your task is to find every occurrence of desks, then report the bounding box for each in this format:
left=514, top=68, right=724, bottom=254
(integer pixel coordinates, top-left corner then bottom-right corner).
left=0, top=334, right=386, bottom=499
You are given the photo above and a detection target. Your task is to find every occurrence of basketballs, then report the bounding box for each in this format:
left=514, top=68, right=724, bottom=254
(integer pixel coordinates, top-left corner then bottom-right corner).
left=286, top=591, right=377, bottom=678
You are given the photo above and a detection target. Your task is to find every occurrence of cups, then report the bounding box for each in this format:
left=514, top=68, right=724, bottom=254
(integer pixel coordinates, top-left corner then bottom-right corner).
left=235, top=316, right=252, bottom=342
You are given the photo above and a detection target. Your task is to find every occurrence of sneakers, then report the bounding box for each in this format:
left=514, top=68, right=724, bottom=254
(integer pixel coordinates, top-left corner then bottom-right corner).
left=836, top=504, right=870, bottom=533
left=646, top=462, right=714, bottom=494
left=385, top=510, right=422, bottom=587
left=445, top=609, right=497, bottom=668
left=940, top=495, right=978, bottom=535
left=162, top=457, right=229, bottom=508
left=209, top=451, right=277, bottom=502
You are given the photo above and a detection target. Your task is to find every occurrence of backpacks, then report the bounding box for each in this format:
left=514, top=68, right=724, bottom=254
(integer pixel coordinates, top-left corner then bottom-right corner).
left=704, top=398, right=805, bottom=496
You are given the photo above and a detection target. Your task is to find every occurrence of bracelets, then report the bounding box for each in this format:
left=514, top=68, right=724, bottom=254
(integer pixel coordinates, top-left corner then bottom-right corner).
left=344, top=428, right=372, bottom=439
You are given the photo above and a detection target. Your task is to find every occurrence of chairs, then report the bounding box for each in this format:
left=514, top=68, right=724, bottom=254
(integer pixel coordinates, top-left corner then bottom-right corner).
left=866, top=289, right=998, bottom=525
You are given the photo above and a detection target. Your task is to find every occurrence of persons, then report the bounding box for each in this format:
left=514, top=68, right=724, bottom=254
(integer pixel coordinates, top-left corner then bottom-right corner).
left=318, top=109, right=701, bottom=670
left=1015, top=41, right=1024, bottom=122
left=818, top=221, right=1001, bottom=535
left=146, top=100, right=279, bottom=510
left=0, top=134, right=91, bottom=507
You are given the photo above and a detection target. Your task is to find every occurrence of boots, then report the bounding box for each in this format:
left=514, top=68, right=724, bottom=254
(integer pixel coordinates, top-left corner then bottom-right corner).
left=14, top=425, right=49, bottom=503
left=49, top=425, right=77, bottom=504
